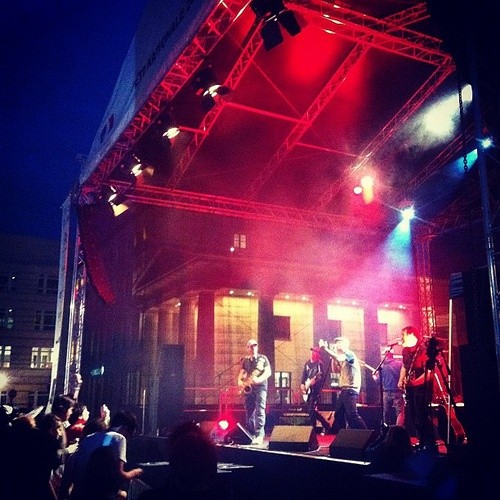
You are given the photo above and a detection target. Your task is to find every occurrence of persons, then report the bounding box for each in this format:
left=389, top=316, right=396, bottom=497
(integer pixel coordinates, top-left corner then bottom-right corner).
left=319, top=337, right=367, bottom=435
left=138, top=424, right=230, bottom=500
left=238, top=340, right=271, bottom=445
left=300, top=347, right=331, bottom=434
left=398, top=323, right=432, bottom=441
left=0, top=389, right=144, bottom=500
left=372, top=348, right=402, bottom=436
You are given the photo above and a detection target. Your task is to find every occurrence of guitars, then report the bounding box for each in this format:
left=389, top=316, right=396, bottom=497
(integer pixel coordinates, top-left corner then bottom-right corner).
left=302, top=371, right=322, bottom=403
left=401, top=371, right=416, bottom=398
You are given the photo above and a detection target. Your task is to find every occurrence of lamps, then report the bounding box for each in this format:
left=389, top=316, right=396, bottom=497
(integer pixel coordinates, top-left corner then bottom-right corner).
left=158, top=117, right=182, bottom=140
left=252, top=0, right=301, bottom=51
left=105, top=185, right=129, bottom=216
left=198, top=73, right=222, bottom=97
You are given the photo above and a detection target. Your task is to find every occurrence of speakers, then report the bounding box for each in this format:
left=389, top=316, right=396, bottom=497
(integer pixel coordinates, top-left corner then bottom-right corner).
left=224, top=423, right=253, bottom=445
left=368, top=426, right=410, bottom=461
left=271, top=425, right=319, bottom=451
left=456, top=265, right=500, bottom=432
left=159, top=343, right=184, bottom=426
left=329, top=429, right=376, bottom=460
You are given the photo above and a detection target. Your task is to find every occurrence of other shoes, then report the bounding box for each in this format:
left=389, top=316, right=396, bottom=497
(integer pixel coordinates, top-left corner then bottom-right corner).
left=251, top=437, right=264, bottom=445
left=321, top=422, right=330, bottom=435
left=412, top=442, right=424, bottom=449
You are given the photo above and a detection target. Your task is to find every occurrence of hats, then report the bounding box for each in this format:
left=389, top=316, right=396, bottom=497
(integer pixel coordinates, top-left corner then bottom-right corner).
left=247, top=339, right=257, bottom=347
left=310, top=348, right=321, bottom=354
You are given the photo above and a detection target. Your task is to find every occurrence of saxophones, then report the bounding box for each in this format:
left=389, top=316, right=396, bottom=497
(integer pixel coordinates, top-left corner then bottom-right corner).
left=237, top=355, right=265, bottom=396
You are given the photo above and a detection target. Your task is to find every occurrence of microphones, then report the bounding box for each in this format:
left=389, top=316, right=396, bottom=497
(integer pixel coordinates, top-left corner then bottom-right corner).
left=388, top=341, right=403, bottom=346
